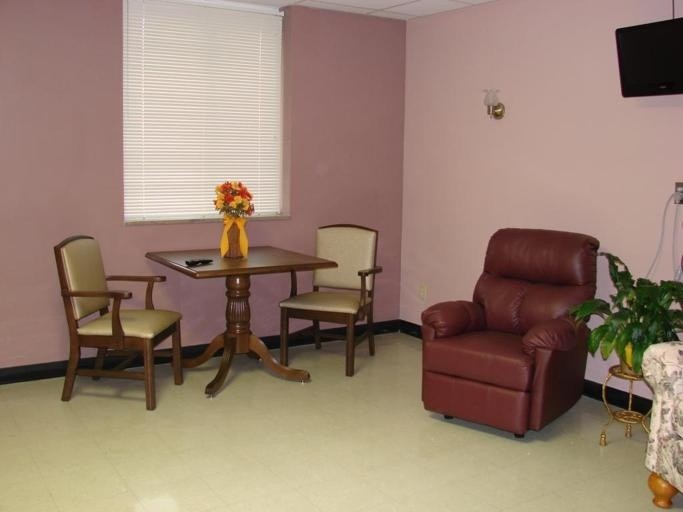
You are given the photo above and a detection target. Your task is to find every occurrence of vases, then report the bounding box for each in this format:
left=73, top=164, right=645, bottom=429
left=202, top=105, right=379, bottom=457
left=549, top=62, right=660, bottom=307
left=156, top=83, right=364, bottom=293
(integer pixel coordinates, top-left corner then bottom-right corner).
left=219, top=216, right=249, bottom=258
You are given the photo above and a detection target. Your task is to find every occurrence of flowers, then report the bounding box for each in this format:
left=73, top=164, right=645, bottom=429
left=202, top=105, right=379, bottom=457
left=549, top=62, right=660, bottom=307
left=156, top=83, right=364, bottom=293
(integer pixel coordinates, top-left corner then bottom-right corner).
left=213, top=181, right=254, bottom=218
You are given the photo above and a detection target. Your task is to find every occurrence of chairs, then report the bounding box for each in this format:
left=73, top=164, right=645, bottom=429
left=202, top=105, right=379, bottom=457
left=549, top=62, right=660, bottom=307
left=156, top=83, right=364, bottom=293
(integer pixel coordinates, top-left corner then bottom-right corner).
left=639, top=341, right=683, bottom=509
left=53, top=234, right=183, bottom=411
left=421, top=227, right=599, bottom=440
left=278, top=223, right=383, bottom=376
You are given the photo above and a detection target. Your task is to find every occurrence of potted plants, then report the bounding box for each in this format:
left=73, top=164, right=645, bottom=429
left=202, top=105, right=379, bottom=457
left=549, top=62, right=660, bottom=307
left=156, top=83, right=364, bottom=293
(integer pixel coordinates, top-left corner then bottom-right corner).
left=570, top=253, right=683, bottom=376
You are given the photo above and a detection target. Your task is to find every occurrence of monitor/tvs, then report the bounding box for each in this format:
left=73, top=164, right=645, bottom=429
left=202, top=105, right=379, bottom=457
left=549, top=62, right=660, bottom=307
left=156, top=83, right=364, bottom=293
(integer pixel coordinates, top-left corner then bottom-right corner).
left=614, top=16, right=683, bottom=97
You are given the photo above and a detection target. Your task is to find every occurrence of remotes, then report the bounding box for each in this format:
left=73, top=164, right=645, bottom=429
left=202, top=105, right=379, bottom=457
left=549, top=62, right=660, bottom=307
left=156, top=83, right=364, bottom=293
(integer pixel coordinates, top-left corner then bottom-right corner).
left=186, top=259, right=213, bottom=266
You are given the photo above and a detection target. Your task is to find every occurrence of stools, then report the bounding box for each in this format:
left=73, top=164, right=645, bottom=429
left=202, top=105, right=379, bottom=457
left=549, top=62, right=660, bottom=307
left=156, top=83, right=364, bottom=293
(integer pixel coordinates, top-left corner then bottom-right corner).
left=600, top=366, right=655, bottom=446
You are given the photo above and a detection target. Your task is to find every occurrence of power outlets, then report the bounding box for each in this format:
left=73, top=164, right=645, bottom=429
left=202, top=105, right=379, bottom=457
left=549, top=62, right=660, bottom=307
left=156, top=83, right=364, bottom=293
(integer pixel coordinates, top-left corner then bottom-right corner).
left=674, top=183, right=683, bottom=204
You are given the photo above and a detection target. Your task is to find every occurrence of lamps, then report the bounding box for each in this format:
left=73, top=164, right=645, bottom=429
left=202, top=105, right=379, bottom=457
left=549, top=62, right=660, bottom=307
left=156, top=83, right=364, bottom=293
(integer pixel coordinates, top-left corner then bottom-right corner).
left=482, top=89, right=505, bottom=121
